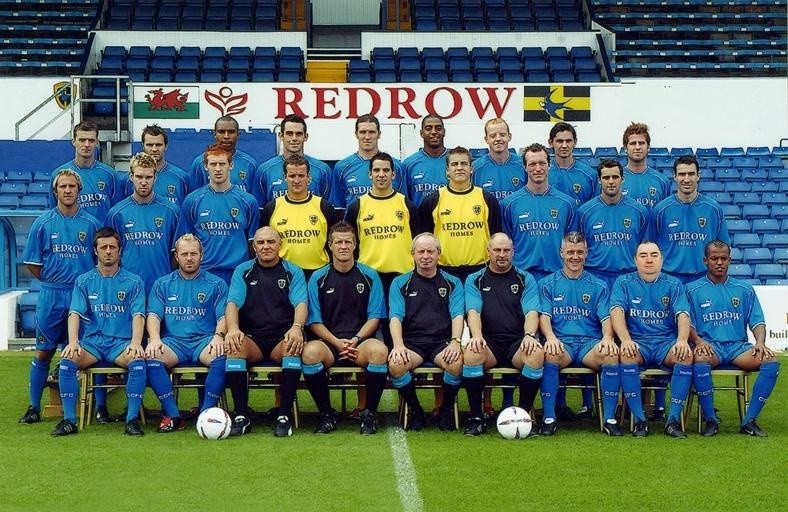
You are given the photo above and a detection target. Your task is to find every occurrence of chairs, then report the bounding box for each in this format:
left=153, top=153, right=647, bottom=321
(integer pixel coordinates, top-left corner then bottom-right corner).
left=0, top=1, right=98, bottom=76
left=0, top=171, right=51, bottom=210
left=17, top=291, right=43, bottom=338
left=28, top=278, right=42, bottom=294
left=594, top=0, right=784, bottom=75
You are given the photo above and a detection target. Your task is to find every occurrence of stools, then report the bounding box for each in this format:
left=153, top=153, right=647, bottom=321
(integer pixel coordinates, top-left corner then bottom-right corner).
left=167, top=363, right=224, bottom=415
left=683, top=365, right=751, bottom=434
left=618, top=368, right=686, bottom=435
left=323, top=365, right=367, bottom=390
left=478, top=366, right=537, bottom=428
left=558, top=366, right=604, bottom=432
left=76, top=367, right=146, bottom=429
left=396, top=366, right=460, bottom=430
left=246, top=364, right=299, bottom=430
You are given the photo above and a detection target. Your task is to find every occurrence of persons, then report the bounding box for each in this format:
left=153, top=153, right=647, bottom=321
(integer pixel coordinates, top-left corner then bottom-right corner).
left=15, top=117, right=781, bottom=437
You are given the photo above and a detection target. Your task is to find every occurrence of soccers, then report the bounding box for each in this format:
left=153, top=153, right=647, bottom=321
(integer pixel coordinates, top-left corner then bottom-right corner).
left=196, top=407, right=231, bottom=439
left=496, top=406, right=532, bottom=439
